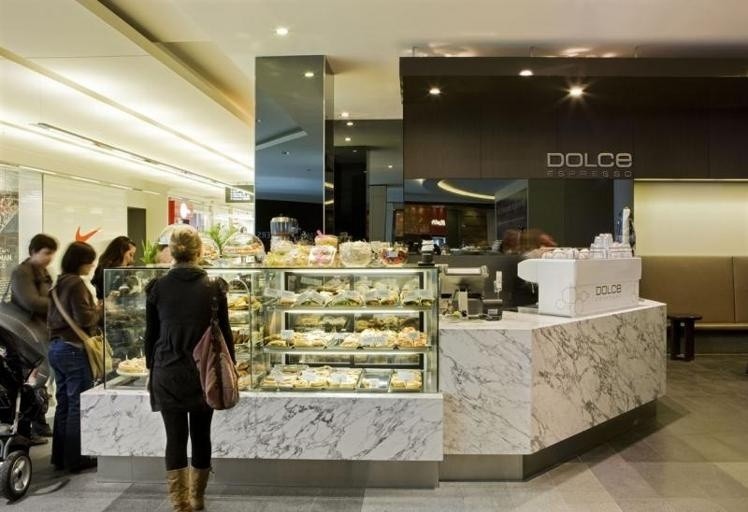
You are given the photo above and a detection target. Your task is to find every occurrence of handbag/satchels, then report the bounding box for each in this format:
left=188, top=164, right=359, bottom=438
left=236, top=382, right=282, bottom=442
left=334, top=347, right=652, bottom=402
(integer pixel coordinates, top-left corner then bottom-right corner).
left=191, top=324, right=239, bottom=409
left=82, top=334, right=119, bottom=380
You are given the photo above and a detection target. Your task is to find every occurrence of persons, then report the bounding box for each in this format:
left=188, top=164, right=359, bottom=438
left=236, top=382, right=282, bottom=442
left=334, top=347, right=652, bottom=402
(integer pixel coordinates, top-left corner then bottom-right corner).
left=124, top=230, right=174, bottom=355
left=92, top=235, right=142, bottom=388
left=500, top=228, right=559, bottom=303
left=8, top=232, right=60, bottom=444
left=145, top=224, right=237, bottom=512
left=49, top=240, right=104, bottom=472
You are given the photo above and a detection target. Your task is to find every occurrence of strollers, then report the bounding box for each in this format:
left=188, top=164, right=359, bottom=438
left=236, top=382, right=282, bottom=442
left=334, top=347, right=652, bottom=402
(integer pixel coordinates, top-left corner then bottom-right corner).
left=0, top=299, right=53, bottom=502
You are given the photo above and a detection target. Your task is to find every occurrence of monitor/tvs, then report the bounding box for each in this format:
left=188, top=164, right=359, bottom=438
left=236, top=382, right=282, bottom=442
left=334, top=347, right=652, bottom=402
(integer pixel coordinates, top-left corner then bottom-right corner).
left=437, top=263, right=488, bottom=299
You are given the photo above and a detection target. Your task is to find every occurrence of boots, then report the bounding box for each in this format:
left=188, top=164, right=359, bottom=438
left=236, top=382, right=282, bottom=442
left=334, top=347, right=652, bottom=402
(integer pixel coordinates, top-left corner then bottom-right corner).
left=165, top=466, right=191, bottom=511
left=190, top=465, right=211, bottom=511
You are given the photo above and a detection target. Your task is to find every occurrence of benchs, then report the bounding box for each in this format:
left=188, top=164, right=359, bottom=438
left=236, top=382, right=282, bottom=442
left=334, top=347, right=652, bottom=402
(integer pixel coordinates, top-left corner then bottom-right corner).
left=638, top=254, right=748, bottom=333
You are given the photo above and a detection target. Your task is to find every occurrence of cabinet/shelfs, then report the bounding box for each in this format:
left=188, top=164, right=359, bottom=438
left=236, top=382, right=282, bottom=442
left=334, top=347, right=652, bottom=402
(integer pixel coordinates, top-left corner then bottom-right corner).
left=101, top=263, right=440, bottom=394
left=494, top=179, right=528, bottom=245
left=461, top=213, right=486, bottom=229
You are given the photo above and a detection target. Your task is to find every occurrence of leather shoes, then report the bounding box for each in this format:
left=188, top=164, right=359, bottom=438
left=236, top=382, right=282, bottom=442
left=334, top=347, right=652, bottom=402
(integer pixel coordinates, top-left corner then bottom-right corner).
left=30, top=439, right=48, bottom=448
left=31, top=429, right=54, bottom=437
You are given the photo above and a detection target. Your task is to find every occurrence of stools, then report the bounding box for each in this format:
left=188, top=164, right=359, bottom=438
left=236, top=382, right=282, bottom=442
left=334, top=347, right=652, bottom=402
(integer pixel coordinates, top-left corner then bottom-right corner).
left=667, top=312, right=702, bottom=360
